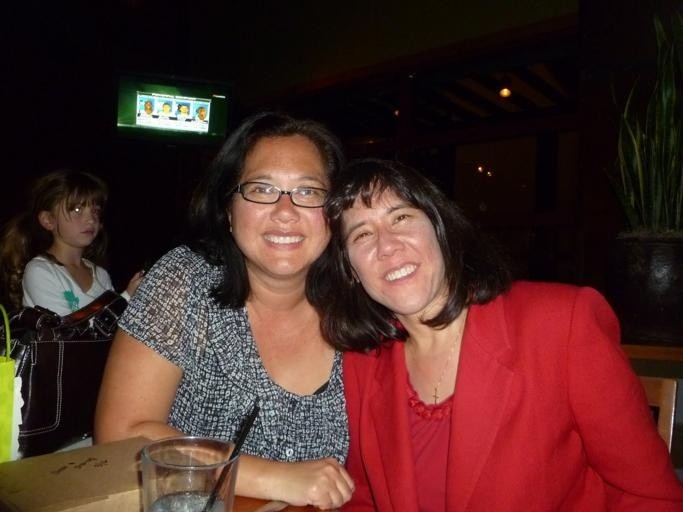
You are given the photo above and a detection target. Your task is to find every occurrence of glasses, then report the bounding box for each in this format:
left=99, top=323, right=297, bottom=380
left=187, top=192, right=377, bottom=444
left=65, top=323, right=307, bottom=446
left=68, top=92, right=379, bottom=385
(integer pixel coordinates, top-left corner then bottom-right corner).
left=228, top=181, right=331, bottom=209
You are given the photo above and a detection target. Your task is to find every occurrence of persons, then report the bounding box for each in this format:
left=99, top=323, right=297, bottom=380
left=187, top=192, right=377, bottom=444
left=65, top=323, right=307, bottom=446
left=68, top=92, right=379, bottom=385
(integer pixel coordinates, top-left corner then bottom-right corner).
left=320, top=152, right=683, bottom=512
left=138, top=101, right=209, bottom=124
left=94, top=111, right=356, bottom=512
left=2, top=167, right=145, bottom=318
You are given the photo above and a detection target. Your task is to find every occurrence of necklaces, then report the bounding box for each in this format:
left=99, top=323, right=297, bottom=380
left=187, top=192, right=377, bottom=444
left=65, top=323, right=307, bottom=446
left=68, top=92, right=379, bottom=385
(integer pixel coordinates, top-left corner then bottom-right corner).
left=408, top=315, right=465, bottom=405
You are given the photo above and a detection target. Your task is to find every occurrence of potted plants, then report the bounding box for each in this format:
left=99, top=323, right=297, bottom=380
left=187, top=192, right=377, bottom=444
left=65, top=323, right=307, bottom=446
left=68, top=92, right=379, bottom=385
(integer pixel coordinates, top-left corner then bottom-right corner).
left=608, top=14, right=681, bottom=343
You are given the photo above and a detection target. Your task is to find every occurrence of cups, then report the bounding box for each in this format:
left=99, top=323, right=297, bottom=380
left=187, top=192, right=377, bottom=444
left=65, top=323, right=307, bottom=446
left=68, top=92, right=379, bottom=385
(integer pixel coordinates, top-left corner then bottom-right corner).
left=138, top=436, right=237, bottom=511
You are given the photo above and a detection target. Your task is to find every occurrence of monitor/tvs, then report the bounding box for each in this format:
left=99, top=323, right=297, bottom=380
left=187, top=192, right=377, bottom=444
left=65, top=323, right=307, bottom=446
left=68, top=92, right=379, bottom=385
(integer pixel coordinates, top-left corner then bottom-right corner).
left=112, top=78, right=230, bottom=143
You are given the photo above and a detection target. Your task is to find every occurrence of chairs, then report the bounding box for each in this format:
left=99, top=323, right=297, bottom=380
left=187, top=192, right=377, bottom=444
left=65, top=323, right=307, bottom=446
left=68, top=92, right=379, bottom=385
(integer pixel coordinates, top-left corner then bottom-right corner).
left=627, top=373, right=676, bottom=459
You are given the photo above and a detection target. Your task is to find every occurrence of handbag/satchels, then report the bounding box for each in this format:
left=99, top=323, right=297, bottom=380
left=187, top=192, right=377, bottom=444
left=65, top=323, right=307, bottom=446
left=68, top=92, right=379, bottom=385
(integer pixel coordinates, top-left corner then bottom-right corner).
left=0, top=290, right=127, bottom=459
